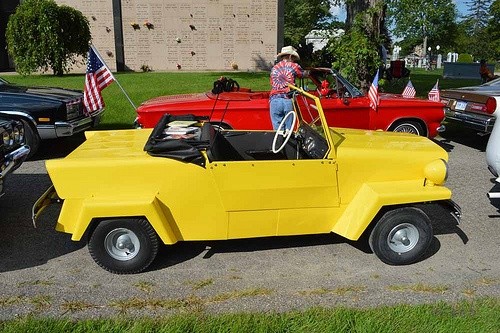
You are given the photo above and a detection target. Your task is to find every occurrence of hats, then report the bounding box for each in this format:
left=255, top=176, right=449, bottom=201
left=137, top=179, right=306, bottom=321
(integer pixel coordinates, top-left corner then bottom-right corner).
left=276, top=46, right=300, bottom=61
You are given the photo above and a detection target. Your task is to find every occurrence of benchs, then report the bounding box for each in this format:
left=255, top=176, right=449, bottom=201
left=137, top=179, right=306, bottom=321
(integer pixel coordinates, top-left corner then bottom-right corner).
left=442, top=61, right=495, bottom=84
left=200, top=124, right=255, bottom=162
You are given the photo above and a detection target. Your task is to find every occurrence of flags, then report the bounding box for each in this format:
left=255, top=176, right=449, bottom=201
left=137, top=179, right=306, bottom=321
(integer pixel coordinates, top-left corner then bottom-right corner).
left=368, top=69, right=379, bottom=112
left=83, top=47, right=115, bottom=118
left=402, top=80, right=416, bottom=96
left=428, top=82, right=439, bottom=102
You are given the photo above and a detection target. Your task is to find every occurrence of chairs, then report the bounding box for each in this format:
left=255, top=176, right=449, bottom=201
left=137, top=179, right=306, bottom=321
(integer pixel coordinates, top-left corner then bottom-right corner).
left=385, top=61, right=411, bottom=91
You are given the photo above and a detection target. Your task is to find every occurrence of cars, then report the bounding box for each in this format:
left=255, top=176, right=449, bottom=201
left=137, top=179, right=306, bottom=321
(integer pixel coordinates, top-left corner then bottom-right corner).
left=0, top=78, right=106, bottom=138
left=435, top=79, right=500, bottom=135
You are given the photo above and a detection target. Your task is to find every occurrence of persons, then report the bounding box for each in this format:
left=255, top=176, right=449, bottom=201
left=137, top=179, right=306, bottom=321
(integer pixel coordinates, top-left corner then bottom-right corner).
left=479, top=59, right=497, bottom=79
left=268, top=46, right=304, bottom=130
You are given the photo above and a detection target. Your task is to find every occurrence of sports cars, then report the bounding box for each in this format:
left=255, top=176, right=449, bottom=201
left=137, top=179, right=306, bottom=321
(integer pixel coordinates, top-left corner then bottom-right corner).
left=135, top=66, right=448, bottom=139
left=31, top=83, right=465, bottom=273
